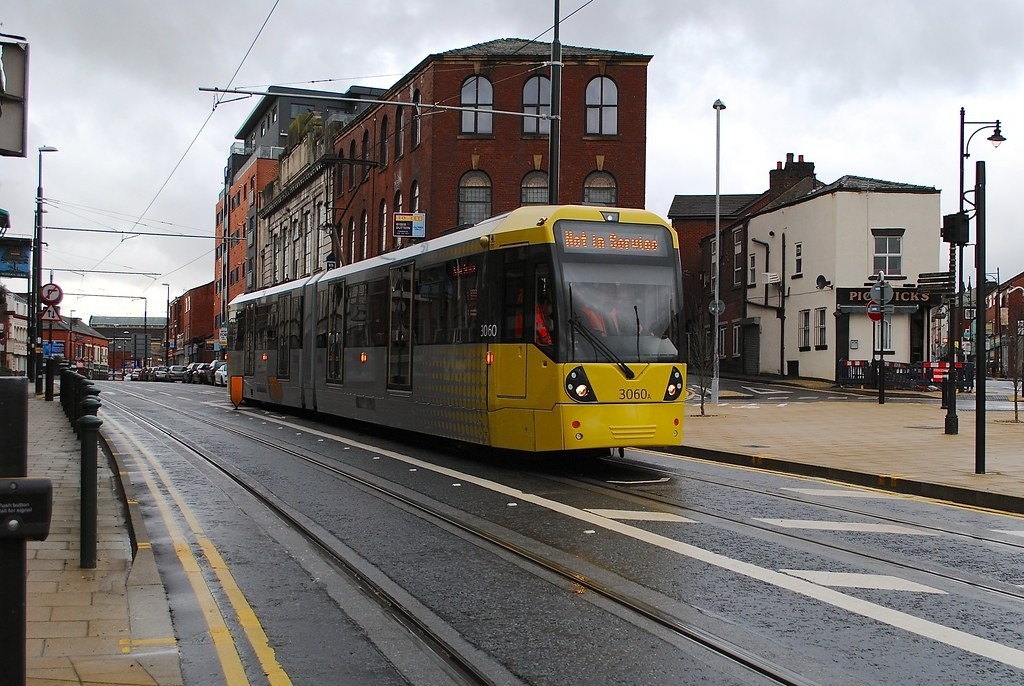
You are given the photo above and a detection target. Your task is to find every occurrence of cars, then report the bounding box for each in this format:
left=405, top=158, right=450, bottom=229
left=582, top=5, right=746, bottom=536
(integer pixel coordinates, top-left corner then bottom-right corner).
left=130, top=359, right=228, bottom=387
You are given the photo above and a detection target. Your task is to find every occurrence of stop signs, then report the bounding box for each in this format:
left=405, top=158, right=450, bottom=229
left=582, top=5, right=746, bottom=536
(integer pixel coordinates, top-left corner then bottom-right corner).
left=866, top=300, right=883, bottom=322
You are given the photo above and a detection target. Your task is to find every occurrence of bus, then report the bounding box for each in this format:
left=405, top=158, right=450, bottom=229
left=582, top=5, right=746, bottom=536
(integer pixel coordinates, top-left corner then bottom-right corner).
left=224, top=155, right=689, bottom=466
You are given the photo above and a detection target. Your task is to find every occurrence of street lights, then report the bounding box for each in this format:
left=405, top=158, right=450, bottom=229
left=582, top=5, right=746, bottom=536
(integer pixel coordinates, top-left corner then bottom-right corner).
left=141, top=297, right=148, bottom=367
left=33, top=145, right=57, bottom=394
left=123, top=330, right=130, bottom=380
left=938, top=106, right=1006, bottom=436
left=162, top=283, right=170, bottom=368
left=707, top=97, right=727, bottom=405
left=69, top=309, right=76, bottom=368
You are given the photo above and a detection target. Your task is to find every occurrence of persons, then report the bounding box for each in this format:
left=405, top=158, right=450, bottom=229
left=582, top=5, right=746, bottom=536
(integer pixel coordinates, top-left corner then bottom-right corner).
left=577, top=283, right=646, bottom=336
left=931, top=352, right=948, bottom=362
left=514, top=279, right=554, bottom=345
